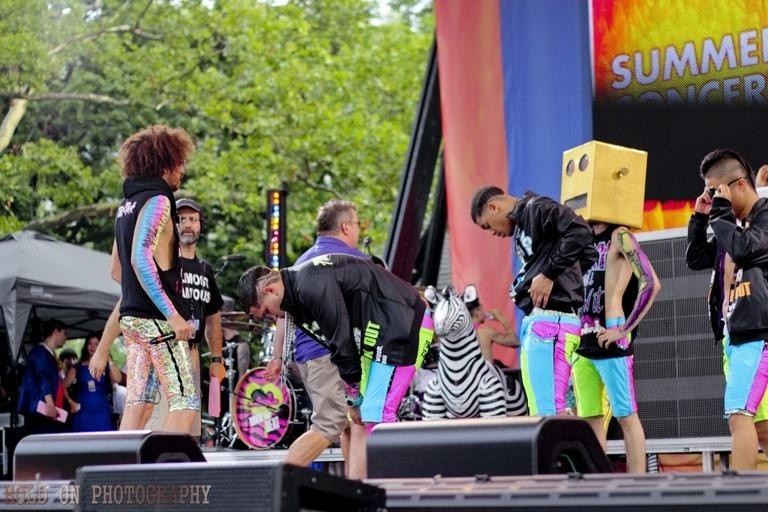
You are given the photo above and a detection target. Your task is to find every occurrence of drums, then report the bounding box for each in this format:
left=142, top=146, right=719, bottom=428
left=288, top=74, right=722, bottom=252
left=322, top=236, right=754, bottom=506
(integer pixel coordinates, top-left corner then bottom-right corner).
left=232, top=367, right=311, bottom=452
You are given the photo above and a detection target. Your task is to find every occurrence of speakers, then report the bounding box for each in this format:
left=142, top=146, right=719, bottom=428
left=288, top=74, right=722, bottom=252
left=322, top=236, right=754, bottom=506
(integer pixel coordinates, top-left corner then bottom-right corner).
left=13, top=430, right=207, bottom=480
left=367, top=416, right=614, bottom=478
left=78, top=462, right=386, bottom=512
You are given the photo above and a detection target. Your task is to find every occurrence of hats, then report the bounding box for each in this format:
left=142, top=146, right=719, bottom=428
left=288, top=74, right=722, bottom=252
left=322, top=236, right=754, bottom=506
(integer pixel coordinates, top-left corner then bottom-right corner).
left=175, top=198, right=201, bottom=213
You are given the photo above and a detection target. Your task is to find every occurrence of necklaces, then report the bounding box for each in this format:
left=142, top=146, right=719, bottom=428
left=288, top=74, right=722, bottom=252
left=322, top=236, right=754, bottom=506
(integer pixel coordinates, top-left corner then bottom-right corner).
left=180, top=251, right=203, bottom=321
left=281, top=310, right=294, bottom=387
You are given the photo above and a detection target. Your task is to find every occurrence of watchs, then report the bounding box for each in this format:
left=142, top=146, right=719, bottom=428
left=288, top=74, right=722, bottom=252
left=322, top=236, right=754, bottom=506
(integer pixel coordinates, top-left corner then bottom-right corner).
left=345, top=393, right=363, bottom=408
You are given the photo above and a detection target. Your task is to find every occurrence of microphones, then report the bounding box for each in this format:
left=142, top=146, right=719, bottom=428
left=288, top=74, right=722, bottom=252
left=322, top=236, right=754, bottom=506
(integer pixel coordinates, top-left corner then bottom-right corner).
left=364, top=236, right=373, bottom=248
left=221, top=256, right=247, bottom=262
left=149, top=320, right=196, bottom=345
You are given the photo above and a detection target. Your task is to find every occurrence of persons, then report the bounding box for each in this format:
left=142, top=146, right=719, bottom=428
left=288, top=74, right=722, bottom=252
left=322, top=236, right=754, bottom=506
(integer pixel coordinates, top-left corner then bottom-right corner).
left=112, top=125, right=201, bottom=434
left=466, top=296, right=520, bottom=365
left=572, top=217, right=661, bottom=474
left=685, top=149, right=768, bottom=470
left=238, top=253, right=434, bottom=431
left=211, top=323, right=250, bottom=441
left=88, top=198, right=227, bottom=429
left=263, top=200, right=367, bottom=485
left=0, top=318, right=122, bottom=432
left=471, top=186, right=599, bottom=418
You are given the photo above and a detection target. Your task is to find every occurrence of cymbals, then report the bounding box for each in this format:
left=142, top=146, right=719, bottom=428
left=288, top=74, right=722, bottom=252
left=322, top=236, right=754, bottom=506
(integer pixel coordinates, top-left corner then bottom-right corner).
left=221, top=311, right=244, bottom=319
left=223, top=322, right=266, bottom=333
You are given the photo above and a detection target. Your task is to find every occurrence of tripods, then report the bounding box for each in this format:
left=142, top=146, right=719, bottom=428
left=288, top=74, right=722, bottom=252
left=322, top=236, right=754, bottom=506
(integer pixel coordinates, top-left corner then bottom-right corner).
left=201, top=330, right=252, bottom=450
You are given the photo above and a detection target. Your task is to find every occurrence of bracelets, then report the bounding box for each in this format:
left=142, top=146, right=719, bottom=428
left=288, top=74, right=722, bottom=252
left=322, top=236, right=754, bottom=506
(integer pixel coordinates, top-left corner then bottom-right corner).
left=269, top=355, right=282, bottom=361
left=209, top=356, right=223, bottom=362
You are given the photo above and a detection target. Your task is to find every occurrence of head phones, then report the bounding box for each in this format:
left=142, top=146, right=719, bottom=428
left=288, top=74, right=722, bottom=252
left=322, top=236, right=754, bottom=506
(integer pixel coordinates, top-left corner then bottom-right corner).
left=199, top=205, right=207, bottom=235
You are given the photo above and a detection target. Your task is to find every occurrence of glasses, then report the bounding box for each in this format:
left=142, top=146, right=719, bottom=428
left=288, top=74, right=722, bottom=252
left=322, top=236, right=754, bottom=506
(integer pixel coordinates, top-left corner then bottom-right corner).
left=180, top=216, right=201, bottom=225
left=704, top=177, right=747, bottom=200
